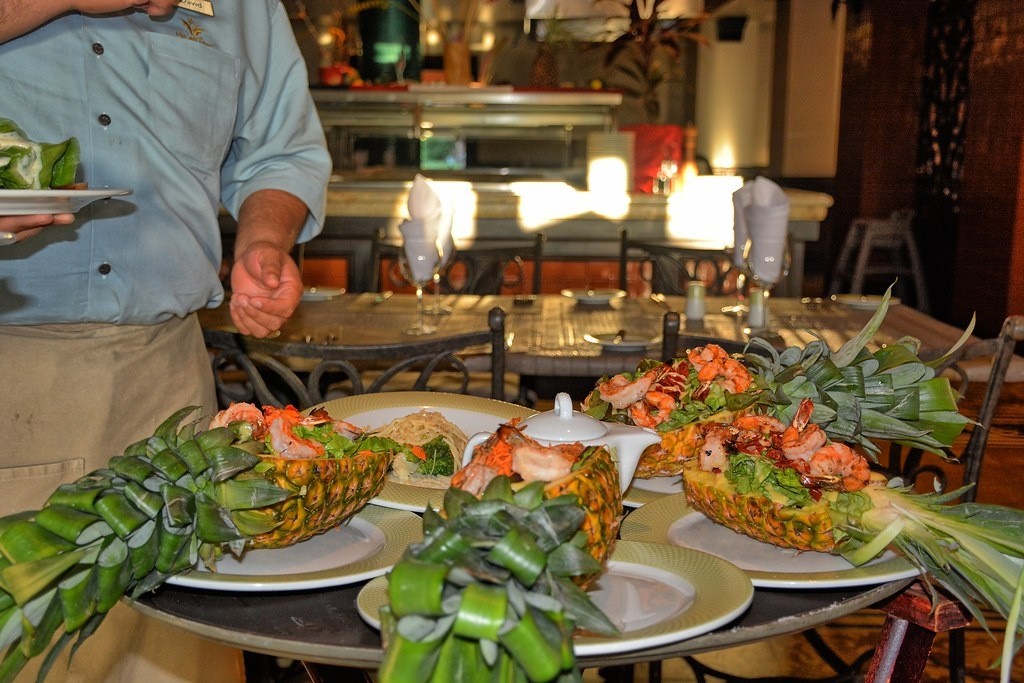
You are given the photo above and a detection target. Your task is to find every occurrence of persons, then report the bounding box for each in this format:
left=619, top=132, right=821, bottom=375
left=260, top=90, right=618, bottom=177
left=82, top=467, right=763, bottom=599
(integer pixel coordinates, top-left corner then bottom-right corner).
left=0, top=0, right=332, bottom=682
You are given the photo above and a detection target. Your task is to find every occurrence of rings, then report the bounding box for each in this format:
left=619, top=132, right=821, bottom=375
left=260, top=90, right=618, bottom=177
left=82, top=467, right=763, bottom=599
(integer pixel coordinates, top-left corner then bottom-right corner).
left=0, top=231, right=17, bottom=246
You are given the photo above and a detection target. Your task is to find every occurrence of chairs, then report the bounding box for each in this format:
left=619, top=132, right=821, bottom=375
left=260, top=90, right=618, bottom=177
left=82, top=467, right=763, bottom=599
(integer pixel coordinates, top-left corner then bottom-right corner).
left=202, top=208, right=1024, bottom=683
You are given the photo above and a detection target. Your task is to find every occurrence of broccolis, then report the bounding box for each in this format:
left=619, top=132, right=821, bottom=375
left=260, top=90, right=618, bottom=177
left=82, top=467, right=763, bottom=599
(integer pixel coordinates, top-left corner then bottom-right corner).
left=400, top=437, right=455, bottom=477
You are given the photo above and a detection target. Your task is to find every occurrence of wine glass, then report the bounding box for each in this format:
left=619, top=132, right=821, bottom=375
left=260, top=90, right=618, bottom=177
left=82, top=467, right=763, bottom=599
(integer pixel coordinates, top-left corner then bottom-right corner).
left=401, top=281, right=437, bottom=335
left=424, top=277, right=452, bottom=316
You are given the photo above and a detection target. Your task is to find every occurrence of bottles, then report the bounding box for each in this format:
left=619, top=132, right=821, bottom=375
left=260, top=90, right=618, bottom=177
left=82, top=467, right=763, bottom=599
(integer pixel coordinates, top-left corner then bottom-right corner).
left=686, top=281, right=705, bottom=319
left=749, top=288, right=769, bottom=328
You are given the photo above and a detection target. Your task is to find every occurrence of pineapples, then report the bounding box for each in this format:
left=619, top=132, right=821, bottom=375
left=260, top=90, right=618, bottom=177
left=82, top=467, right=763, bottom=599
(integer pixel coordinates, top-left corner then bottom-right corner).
left=0, top=399, right=391, bottom=682
left=681, top=455, right=1023, bottom=649
left=374, top=450, right=623, bottom=683
left=636, top=277, right=980, bottom=479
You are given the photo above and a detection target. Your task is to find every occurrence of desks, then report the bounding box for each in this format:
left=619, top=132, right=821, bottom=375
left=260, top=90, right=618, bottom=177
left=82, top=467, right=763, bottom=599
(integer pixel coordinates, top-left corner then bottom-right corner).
left=310, top=80, right=620, bottom=174
left=312, top=170, right=837, bottom=298
left=199, top=289, right=1024, bottom=418
left=114, top=507, right=917, bottom=683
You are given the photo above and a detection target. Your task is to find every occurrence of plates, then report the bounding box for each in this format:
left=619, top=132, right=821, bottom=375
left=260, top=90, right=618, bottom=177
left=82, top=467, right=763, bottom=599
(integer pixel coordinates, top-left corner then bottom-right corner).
left=620, top=474, right=928, bottom=588
left=300, top=286, right=346, bottom=301
left=356, top=539, right=754, bottom=656
left=583, top=333, right=662, bottom=352
left=622, top=470, right=685, bottom=508
left=0, top=187, right=134, bottom=215
left=561, top=287, right=627, bottom=305
left=164, top=504, right=425, bottom=591
left=300, top=391, right=541, bottom=513
left=835, top=295, right=901, bottom=310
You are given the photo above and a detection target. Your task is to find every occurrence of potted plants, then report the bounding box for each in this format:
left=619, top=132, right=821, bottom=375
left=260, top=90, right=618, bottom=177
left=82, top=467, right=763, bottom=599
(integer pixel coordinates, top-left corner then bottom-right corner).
left=283, top=0, right=713, bottom=194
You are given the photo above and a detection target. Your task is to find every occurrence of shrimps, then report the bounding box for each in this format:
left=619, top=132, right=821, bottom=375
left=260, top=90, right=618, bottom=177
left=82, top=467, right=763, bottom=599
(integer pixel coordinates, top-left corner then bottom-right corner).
left=449, top=417, right=575, bottom=499
left=334, top=419, right=369, bottom=441
left=597, top=343, right=752, bottom=430
left=700, top=395, right=872, bottom=495
left=208, top=401, right=326, bottom=459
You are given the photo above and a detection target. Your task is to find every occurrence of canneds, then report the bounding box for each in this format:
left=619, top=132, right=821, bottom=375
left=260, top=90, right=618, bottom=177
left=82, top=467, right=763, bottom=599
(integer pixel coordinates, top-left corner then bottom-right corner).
left=686, top=281, right=706, bottom=320
left=749, top=288, right=769, bottom=327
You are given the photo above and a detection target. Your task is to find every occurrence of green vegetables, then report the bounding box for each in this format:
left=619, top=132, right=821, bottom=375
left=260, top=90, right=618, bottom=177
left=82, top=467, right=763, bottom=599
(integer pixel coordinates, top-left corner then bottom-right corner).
left=581, top=357, right=817, bottom=506
left=258, top=426, right=400, bottom=456
left=0, top=119, right=79, bottom=193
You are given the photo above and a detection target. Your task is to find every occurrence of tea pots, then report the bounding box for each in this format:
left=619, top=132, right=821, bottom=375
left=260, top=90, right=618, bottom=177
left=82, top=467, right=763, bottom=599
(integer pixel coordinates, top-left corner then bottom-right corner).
left=462, top=392, right=662, bottom=494
